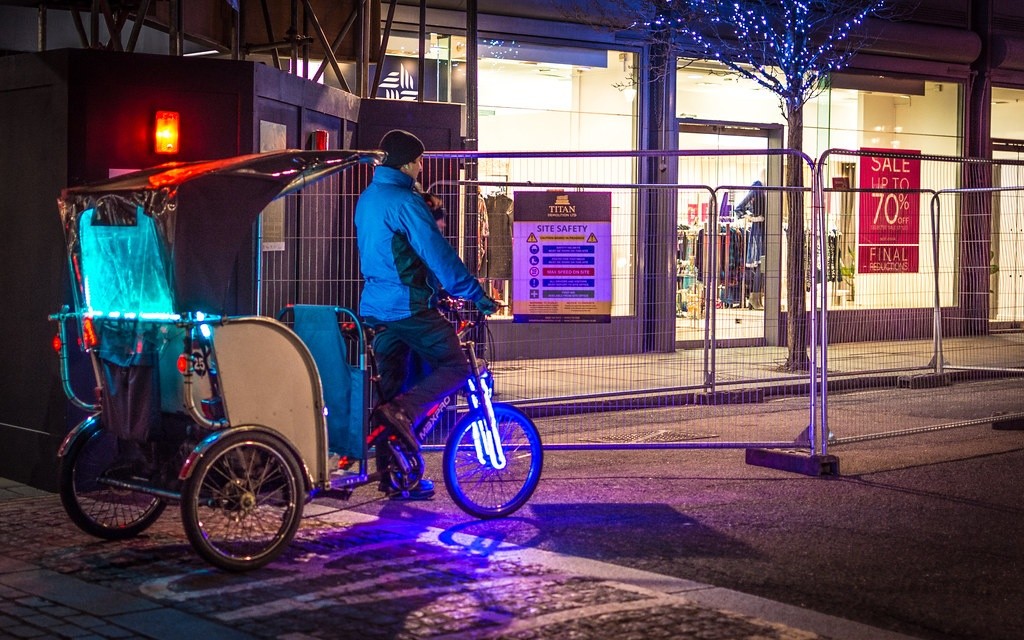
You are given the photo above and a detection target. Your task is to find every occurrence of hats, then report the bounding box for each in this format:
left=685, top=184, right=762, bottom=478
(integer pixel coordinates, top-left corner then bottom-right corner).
left=378, top=129, right=425, bottom=170
left=421, top=192, right=446, bottom=220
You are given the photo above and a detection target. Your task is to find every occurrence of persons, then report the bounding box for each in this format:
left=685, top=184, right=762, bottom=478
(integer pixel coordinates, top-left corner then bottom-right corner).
left=354, top=130, right=496, bottom=499
left=735, top=168, right=767, bottom=310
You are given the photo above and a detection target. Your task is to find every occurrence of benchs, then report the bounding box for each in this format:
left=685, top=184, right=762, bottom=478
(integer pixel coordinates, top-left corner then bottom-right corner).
left=50, top=311, right=322, bottom=442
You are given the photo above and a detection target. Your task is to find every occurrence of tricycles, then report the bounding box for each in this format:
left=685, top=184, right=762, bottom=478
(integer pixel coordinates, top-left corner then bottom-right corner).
left=41, top=147, right=545, bottom=573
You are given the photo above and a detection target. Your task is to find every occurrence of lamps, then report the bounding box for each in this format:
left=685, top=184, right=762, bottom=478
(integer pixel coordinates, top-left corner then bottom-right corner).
left=310, top=129, right=329, bottom=151
left=152, top=108, right=181, bottom=156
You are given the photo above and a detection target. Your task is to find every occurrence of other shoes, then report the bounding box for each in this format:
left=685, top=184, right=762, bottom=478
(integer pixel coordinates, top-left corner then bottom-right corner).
left=378, top=473, right=391, bottom=491
left=374, top=402, right=418, bottom=455
